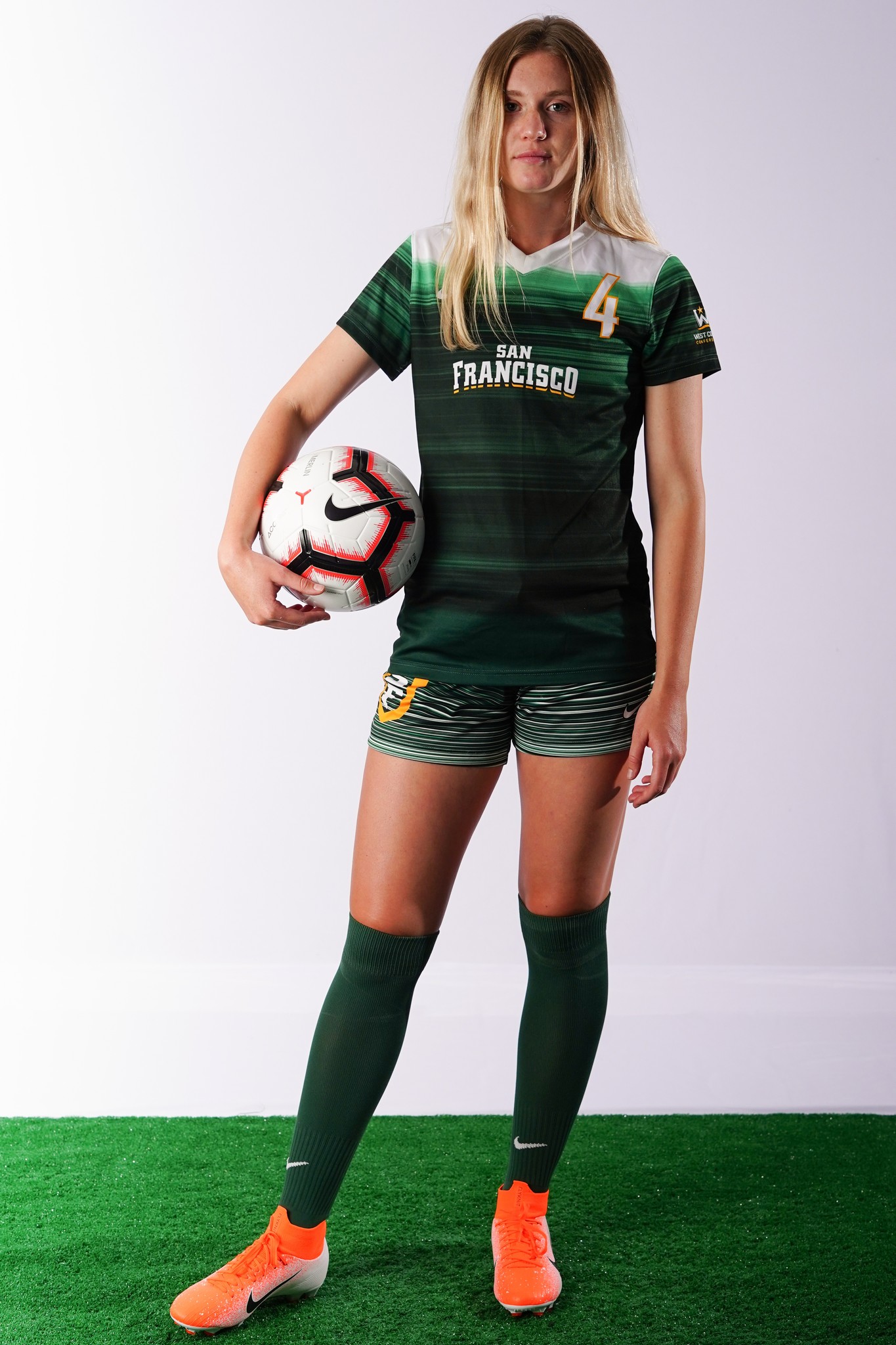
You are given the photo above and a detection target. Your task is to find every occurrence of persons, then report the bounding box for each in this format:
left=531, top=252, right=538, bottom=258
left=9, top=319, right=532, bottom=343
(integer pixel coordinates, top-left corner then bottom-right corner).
left=165, top=14, right=723, bottom=1335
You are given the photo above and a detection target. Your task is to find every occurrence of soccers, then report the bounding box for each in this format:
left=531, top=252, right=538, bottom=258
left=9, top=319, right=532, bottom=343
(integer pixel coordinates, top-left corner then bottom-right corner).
left=259, top=446, right=427, bottom=613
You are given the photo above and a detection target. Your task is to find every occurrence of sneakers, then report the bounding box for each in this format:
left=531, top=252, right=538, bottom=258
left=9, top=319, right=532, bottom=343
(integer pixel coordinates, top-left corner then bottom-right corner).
left=169, top=1206, right=330, bottom=1334
left=488, top=1181, right=563, bottom=1318
